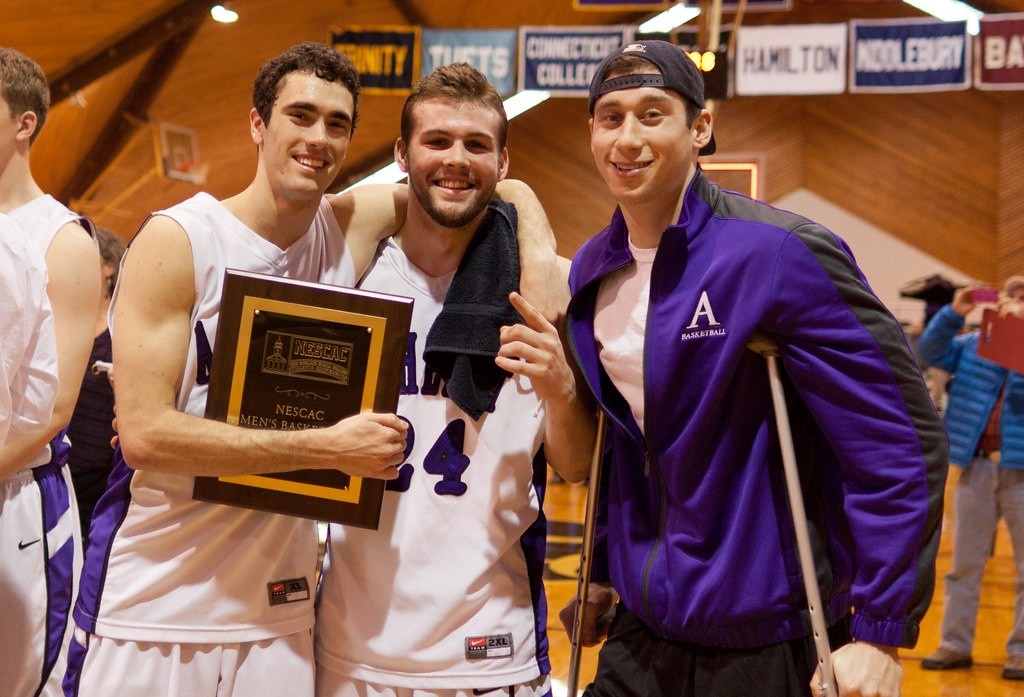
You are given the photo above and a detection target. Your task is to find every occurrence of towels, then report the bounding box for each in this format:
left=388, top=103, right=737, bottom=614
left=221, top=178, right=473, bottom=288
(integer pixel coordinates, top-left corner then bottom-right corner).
left=423, top=197, right=521, bottom=422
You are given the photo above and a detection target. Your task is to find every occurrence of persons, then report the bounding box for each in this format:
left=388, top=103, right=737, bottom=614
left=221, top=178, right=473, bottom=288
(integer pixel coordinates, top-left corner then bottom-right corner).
left=559, top=40, right=951, bottom=697
left=66, top=227, right=125, bottom=561
left=62, top=41, right=563, bottom=697
left=0, top=46, right=103, bottom=697
left=916, top=276, right=1024, bottom=680
left=112, top=61, right=597, bottom=697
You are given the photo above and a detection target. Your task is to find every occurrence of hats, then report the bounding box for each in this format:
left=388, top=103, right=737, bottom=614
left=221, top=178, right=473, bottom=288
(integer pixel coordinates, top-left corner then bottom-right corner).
left=588, top=40, right=716, bottom=156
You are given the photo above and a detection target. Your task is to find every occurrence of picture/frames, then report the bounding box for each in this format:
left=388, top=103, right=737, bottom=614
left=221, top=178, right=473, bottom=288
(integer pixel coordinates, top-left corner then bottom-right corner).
left=190, top=268, right=418, bottom=528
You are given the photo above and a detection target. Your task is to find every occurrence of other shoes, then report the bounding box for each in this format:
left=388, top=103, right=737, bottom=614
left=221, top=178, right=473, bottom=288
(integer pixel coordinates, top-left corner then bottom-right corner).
left=921, top=647, right=973, bottom=670
left=1002, top=668, right=1024, bottom=678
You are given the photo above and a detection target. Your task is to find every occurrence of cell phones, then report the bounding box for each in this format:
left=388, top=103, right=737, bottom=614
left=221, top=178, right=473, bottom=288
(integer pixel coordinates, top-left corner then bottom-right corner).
left=973, top=288, right=999, bottom=304
